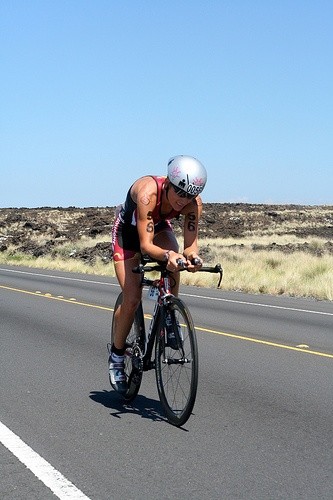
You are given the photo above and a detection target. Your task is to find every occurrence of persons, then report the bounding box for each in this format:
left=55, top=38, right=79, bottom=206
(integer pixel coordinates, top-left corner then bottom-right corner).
left=107, top=155, right=207, bottom=393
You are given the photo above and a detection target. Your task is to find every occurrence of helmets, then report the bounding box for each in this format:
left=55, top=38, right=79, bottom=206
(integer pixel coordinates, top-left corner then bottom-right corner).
left=167, top=155, right=207, bottom=195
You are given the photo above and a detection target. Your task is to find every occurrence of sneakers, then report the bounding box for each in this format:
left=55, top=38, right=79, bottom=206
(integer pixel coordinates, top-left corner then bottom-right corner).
left=108, top=352, right=128, bottom=395
left=165, top=310, right=185, bottom=350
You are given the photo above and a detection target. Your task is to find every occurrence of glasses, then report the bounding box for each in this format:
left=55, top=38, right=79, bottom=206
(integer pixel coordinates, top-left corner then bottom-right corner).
left=167, top=182, right=196, bottom=200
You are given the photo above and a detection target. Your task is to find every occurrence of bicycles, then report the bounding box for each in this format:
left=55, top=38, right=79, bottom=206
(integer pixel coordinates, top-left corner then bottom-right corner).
left=106, top=255, right=224, bottom=428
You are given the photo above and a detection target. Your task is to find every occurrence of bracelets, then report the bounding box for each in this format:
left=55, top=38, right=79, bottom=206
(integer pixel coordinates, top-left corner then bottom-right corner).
left=163, top=250, right=173, bottom=262
